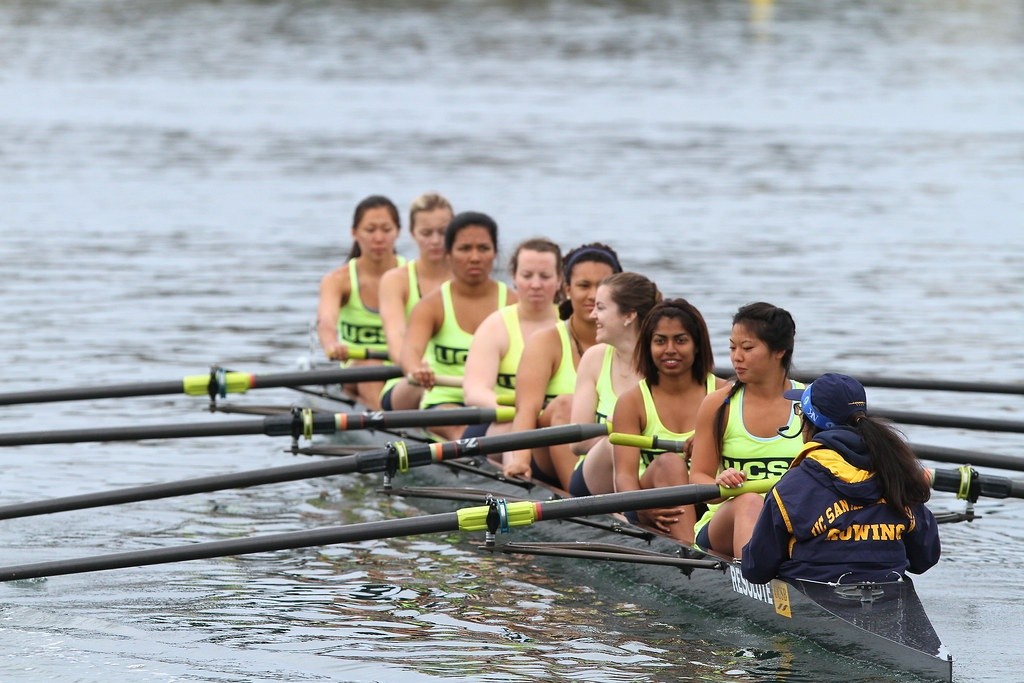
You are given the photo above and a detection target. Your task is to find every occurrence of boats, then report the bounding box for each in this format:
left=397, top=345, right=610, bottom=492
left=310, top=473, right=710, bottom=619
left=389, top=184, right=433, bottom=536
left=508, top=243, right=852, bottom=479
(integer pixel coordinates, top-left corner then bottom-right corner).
left=274, top=359, right=954, bottom=683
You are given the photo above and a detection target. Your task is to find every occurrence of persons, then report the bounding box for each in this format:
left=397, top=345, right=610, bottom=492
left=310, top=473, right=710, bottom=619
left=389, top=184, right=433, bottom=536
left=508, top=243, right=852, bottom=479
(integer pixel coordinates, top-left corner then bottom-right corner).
left=568, top=271, right=663, bottom=496
left=742, top=373, right=941, bottom=586
left=612, top=297, right=728, bottom=545
left=501, top=243, right=623, bottom=492
left=377, top=193, right=457, bottom=410
left=461, top=238, right=567, bottom=462
left=315, top=194, right=412, bottom=412
left=687, top=302, right=810, bottom=559
left=397, top=209, right=519, bottom=446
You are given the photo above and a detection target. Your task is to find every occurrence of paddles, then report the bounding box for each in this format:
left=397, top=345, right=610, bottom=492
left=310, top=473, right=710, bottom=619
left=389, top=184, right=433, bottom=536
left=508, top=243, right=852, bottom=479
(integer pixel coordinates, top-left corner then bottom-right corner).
left=0, top=344, right=1024, bottom=583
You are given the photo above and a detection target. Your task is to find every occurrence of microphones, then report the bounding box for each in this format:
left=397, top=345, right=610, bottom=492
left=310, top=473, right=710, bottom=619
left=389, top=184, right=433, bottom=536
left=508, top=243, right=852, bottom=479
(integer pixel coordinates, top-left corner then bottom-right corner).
left=777, top=414, right=807, bottom=438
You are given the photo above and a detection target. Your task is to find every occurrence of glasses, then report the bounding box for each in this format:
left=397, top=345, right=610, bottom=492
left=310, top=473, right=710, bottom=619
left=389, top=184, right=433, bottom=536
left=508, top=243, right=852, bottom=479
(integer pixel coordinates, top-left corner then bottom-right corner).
left=793, top=403, right=802, bottom=415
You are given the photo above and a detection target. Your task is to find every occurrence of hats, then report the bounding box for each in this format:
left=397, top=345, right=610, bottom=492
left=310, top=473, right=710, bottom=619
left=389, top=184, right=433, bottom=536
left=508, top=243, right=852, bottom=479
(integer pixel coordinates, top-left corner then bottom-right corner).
left=783, top=372, right=867, bottom=430
